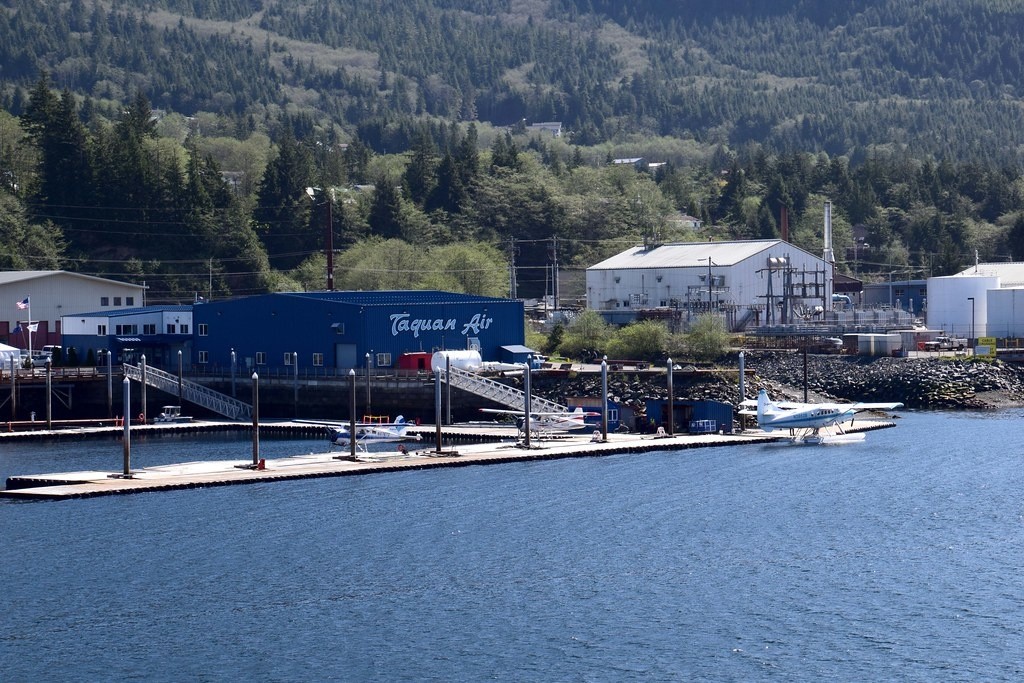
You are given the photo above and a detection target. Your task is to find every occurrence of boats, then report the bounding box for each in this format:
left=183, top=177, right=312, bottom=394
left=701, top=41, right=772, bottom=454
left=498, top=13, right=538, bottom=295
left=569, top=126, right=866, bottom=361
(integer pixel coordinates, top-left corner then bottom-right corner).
left=154, top=406, right=194, bottom=423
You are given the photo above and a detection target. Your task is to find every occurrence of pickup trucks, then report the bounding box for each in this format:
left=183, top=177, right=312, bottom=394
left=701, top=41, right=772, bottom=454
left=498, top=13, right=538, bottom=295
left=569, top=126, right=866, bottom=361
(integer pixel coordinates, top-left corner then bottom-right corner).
left=925, top=336, right=968, bottom=352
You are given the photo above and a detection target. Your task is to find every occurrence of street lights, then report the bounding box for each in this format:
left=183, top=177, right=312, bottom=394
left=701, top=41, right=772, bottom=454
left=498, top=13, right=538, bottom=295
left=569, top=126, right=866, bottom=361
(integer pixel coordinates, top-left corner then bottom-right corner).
left=968, top=297, right=976, bottom=356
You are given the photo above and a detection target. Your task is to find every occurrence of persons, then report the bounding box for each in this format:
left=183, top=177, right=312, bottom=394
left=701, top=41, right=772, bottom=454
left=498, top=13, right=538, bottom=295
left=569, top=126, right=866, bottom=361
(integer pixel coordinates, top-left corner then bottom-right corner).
left=581, top=348, right=597, bottom=363
left=638, top=416, right=656, bottom=434
left=398, top=445, right=408, bottom=455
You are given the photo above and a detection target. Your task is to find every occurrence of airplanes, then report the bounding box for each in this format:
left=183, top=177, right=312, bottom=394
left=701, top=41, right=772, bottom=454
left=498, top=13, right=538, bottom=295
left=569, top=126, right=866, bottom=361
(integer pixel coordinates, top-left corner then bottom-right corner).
left=479, top=404, right=601, bottom=436
left=739, top=389, right=906, bottom=444
left=292, top=414, right=420, bottom=446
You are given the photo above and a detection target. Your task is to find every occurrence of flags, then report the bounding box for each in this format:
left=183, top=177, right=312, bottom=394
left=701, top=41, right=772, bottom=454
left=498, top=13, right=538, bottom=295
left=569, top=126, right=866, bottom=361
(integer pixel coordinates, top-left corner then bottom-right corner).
left=12, top=324, right=23, bottom=334
left=16, top=296, right=30, bottom=310
left=27, top=323, right=39, bottom=332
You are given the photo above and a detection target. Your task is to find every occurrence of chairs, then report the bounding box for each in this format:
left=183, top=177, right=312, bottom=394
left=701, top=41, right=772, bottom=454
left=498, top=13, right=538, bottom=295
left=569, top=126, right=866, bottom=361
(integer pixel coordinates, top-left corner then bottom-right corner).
left=656, top=427, right=666, bottom=436
left=592, top=430, right=602, bottom=440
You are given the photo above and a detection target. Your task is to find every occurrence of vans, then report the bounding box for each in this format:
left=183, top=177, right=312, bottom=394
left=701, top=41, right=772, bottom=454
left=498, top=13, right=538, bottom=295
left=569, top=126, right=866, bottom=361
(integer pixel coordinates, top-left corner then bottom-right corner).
left=32, top=355, right=52, bottom=367
left=40, top=345, right=62, bottom=355
